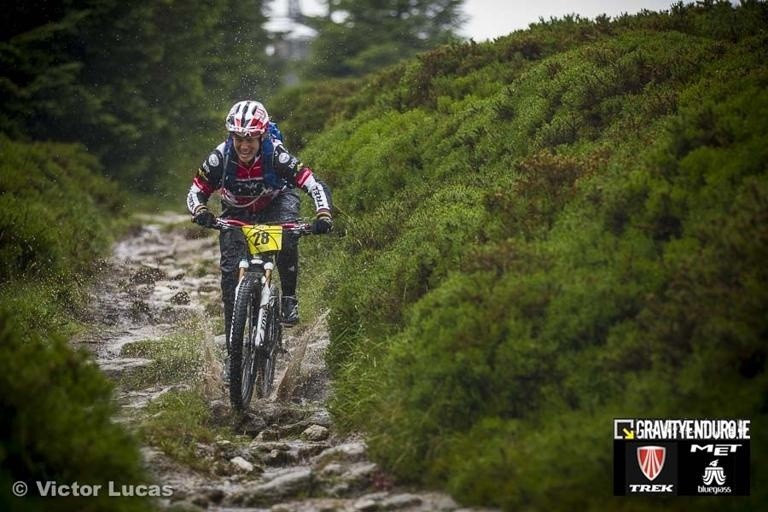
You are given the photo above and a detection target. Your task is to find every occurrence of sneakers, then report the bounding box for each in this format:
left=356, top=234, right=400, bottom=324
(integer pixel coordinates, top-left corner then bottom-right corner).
left=280, top=295, right=299, bottom=325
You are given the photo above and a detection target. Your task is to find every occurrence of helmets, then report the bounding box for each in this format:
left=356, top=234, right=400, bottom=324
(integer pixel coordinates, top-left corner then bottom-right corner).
left=225, top=101, right=269, bottom=137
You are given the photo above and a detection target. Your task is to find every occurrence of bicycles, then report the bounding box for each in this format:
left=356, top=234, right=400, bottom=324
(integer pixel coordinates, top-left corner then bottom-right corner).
left=190, top=212, right=335, bottom=414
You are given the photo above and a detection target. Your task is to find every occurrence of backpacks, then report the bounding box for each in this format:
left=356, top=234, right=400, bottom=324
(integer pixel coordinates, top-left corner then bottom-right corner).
left=263, top=122, right=282, bottom=174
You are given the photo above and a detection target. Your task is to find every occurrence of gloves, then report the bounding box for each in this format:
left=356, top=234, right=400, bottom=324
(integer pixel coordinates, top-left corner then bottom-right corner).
left=313, top=220, right=332, bottom=235
left=195, top=210, right=216, bottom=228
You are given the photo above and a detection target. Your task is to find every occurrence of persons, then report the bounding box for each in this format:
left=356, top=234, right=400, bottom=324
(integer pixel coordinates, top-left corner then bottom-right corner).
left=186, top=100, right=333, bottom=386
